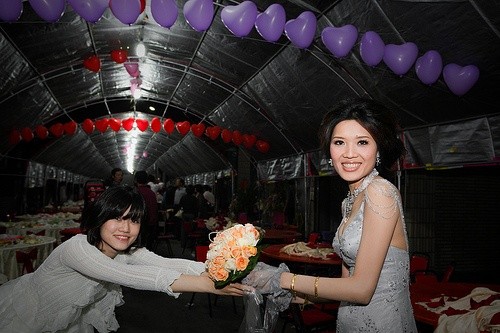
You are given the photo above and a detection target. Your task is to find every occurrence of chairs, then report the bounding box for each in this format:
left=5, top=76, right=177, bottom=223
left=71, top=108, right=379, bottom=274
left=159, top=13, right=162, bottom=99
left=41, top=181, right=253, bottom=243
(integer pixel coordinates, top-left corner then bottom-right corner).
left=408, top=251, right=453, bottom=293
left=281, top=293, right=339, bottom=333
left=272, top=212, right=284, bottom=225
left=309, top=232, right=319, bottom=243
left=164, top=210, right=237, bottom=318
left=238, top=212, right=248, bottom=222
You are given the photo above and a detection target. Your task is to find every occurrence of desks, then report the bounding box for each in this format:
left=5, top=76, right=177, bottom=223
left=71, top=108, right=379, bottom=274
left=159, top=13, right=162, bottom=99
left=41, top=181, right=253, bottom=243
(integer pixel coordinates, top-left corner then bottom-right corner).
left=0, top=205, right=85, bottom=284
left=409, top=282, right=500, bottom=333
left=264, top=229, right=302, bottom=239
left=263, top=243, right=342, bottom=275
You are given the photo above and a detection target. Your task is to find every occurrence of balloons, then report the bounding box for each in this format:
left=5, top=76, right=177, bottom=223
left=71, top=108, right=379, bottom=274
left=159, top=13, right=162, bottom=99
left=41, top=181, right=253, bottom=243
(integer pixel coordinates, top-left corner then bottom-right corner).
left=10, top=121, right=78, bottom=144
left=83, top=55, right=101, bottom=73
left=136, top=117, right=191, bottom=136
left=111, top=49, right=127, bottom=62
left=191, top=124, right=232, bottom=143
left=0, top=0, right=216, bottom=32
left=232, top=130, right=270, bottom=153
left=220, top=1, right=385, bottom=68
left=383, top=42, right=479, bottom=97
left=125, top=63, right=139, bottom=77
left=82, top=118, right=134, bottom=134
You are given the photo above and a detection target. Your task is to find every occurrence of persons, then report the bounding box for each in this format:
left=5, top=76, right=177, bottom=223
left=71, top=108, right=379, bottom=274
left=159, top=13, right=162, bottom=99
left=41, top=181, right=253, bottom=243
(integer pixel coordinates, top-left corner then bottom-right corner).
left=0, top=184, right=256, bottom=333
left=249, top=97, right=418, bottom=333
left=132, top=171, right=158, bottom=248
left=148, top=175, right=215, bottom=241
left=104, top=168, right=123, bottom=187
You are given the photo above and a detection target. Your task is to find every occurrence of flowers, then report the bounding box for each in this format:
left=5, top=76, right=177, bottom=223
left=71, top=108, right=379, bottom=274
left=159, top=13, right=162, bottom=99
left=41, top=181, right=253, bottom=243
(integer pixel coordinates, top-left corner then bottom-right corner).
left=203, top=216, right=269, bottom=287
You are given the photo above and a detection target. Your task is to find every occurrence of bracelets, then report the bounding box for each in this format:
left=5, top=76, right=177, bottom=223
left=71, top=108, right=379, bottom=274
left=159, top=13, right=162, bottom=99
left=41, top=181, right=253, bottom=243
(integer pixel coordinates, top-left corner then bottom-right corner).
left=314, top=276, right=320, bottom=296
left=290, top=274, right=297, bottom=291
